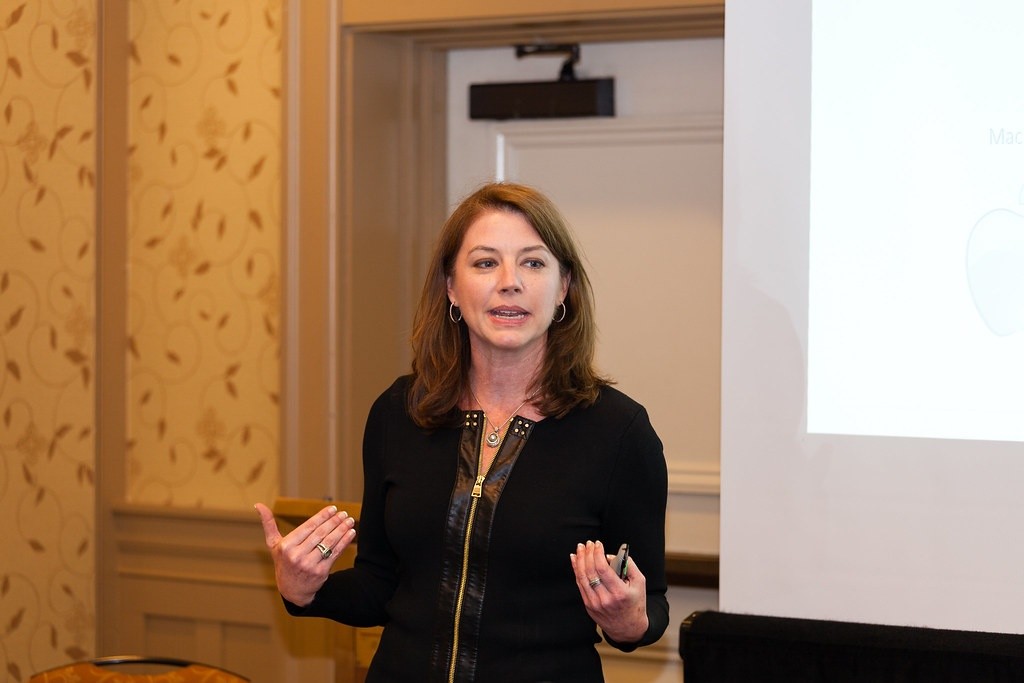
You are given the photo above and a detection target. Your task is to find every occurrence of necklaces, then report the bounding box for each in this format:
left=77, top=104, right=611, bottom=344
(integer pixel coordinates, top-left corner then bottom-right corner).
left=468, top=381, right=541, bottom=446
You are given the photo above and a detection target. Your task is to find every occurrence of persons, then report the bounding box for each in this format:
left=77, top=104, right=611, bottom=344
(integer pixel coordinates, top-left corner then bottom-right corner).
left=251, top=184, right=668, bottom=683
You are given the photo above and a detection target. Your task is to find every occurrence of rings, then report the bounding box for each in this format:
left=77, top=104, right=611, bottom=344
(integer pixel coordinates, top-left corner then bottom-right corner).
left=590, top=578, right=602, bottom=588
left=317, top=544, right=332, bottom=559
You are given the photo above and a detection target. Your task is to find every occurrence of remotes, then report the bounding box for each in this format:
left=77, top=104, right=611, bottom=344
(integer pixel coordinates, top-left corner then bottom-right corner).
left=609, top=543, right=629, bottom=580
